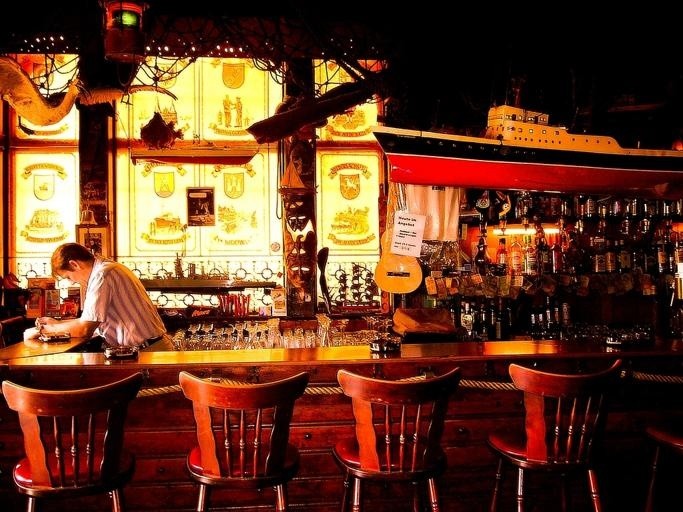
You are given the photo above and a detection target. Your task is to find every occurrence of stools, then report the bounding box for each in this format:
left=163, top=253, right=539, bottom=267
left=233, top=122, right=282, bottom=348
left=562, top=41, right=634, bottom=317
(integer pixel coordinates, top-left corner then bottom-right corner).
left=641, top=421, right=682, bottom=506
left=489, top=360, right=624, bottom=511
left=2, top=370, right=145, bottom=511
left=178, top=368, right=308, bottom=510
left=333, top=365, right=463, bottom=512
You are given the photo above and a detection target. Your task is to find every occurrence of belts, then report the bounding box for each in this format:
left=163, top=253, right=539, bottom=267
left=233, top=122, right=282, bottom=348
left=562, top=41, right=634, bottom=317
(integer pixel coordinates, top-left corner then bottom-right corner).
left=139, top=334, right=164, bottom=351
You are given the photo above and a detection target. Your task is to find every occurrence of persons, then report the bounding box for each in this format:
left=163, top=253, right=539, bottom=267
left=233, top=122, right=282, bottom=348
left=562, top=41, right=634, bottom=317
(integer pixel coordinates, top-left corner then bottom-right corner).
left=21, top=241, right=175, bottom=351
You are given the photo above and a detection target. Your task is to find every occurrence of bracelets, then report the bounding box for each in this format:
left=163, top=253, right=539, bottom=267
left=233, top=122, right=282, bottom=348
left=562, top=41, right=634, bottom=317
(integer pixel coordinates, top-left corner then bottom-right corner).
left=36, top=325, right=44, bottom=337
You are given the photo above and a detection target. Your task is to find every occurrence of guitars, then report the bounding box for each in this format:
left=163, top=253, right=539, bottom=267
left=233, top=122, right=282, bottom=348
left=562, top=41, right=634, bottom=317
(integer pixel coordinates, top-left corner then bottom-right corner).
left=375, top=183, right=423, bottom=295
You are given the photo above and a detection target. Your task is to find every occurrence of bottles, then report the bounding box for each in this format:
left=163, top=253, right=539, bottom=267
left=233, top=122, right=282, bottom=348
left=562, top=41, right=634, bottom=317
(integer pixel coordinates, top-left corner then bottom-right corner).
left=450, top=189, right=683, bottom=341
left=172, top=327, right=317, bottom=351
